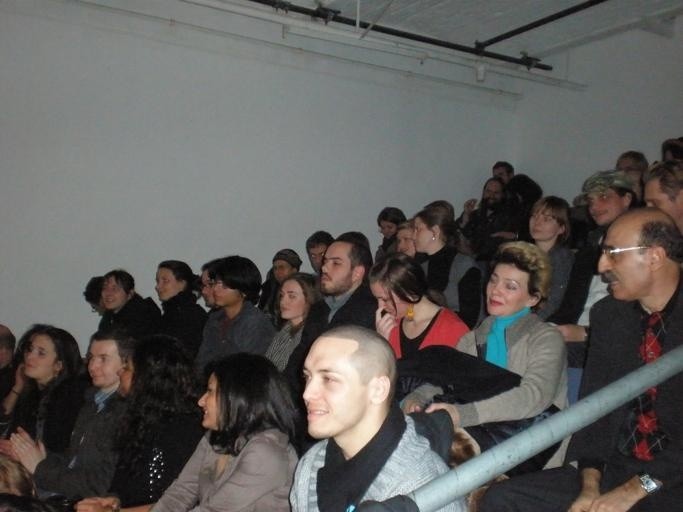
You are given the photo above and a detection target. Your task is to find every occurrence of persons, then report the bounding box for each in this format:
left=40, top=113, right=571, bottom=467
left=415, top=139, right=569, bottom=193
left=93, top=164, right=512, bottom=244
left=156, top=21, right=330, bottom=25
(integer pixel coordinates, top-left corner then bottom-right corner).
left=398, top=240, right=574, bottom=483
left=262, top=247, right=303, bottom=316
left=288, top=323, right=467, bottom=511
left=100, top=267, right=161, bottom=351
left=153, top=258, right=208, bottom=354
left=298, top=237, right=390, bottom=381
left=147, top=352, right=297, bottom=512
left=10, top=329, right=136, bottom=499
left=615, top=150, right=650, bottom=183
left=413, top=200, right=488, bottom=330
left=641, top=161, right=683, bottom=234
left=202, top=254, right=277, bottom=357
left=1, top=325, right=16, bottom=399
left=3, top=323, right=54, bottom=415
left=523, top=195, right=576, bottom=327
left=659, top=139, right=679, bottom=164
left=368, top=251, right=469, bottom=358
left=73, top=333, right=200, bottom=511
left=455, top=178, right=516, bottom=248
left=393, top=221, right=417, bottom=260
left=305, top=230, right=337, bottom=280
left=199, top=258, right=226, bottom=307
left=543, top=170, right=640, bottom=327
left=475, top=206, right=683, bottom=512
left=492, top=161, right=513, bottom=184
left=81, top=275, right=106, bottom=317
left=2, top=326, right=87, bottom=454
left=265, top=271, right=321, bottom=370
left=373, top=207, right=408, bottom=267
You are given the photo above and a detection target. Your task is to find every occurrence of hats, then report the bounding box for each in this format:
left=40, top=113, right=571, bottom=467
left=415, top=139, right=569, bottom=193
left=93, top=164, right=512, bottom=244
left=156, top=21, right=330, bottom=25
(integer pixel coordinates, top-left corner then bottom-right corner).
left=274, top=249, right=302, bottom=271
left=572, top=171, right=642, bottom=207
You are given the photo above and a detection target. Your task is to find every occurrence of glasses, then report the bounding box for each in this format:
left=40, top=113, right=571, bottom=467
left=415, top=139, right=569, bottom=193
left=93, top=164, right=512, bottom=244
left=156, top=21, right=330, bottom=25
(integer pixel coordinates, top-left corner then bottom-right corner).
left=199, top=281, right=216, bottom=289
left=601, top=246, right=649, bottom=260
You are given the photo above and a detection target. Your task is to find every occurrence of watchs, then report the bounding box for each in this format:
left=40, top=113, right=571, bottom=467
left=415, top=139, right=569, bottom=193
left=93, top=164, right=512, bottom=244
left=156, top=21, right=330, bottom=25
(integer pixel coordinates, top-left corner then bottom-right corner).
left=636, top=470, right=659, bottom=493
left=11, top=387, right=17, bottom=394
